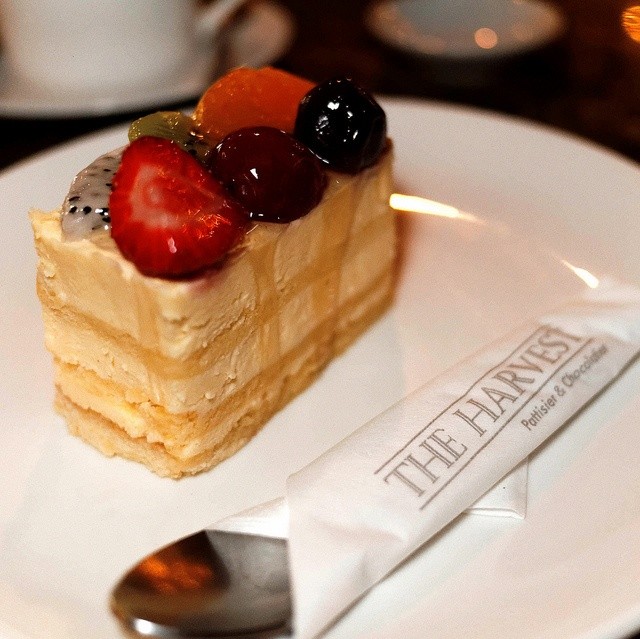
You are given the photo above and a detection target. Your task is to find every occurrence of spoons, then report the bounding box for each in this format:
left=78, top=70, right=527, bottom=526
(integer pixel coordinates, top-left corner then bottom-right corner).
left=110, top=527, right=296, bottom=639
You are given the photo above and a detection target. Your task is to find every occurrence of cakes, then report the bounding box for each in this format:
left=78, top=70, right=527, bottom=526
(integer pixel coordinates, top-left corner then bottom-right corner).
left=30, top=67, right=397, bottom=479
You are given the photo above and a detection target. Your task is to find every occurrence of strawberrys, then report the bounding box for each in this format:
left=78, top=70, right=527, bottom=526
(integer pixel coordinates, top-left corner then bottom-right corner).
left=111, top=134, right=237, bottom=276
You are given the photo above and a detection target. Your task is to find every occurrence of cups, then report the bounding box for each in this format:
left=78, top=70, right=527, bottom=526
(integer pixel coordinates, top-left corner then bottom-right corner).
left=0, top=2, right=249, bottom=105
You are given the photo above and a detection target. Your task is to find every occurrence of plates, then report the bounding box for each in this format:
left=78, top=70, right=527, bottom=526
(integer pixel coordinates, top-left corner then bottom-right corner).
left=0, top=4, right=299, bottom=118
left=0, top=96, right=638, bottom=639
